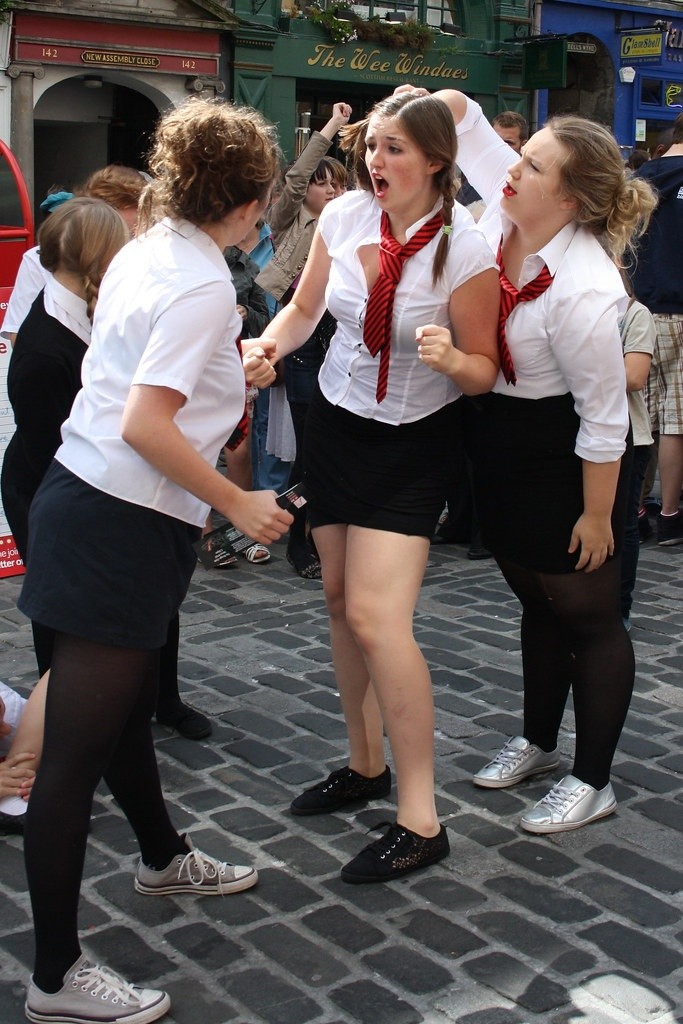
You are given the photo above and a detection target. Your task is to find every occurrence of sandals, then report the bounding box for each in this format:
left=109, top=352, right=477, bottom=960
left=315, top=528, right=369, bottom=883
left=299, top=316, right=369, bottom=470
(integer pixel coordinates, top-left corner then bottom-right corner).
left=215, top=556, right=238, bottom=568
left=245, top=543, right=271, bottom=564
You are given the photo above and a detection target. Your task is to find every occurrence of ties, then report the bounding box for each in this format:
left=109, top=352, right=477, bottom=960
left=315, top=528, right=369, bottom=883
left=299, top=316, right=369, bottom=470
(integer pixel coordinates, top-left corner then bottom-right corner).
left=362, top=208, right=448, bottom=405
left=225, top=337, right=250, bottom=453
left=495, top=233, right=556, bottom=388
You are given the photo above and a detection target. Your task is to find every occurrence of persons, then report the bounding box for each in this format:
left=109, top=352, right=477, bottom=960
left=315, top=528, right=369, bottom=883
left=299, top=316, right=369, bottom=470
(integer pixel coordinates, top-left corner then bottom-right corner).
left=205, top=103, right=350, bottom=579
left=390, top=84, right=657, bottom=833
left=619, top=111, right=683, bottom=546
left=0, top=105, right=293, bottom=1024
left=245, top=92, right=501, bottom=885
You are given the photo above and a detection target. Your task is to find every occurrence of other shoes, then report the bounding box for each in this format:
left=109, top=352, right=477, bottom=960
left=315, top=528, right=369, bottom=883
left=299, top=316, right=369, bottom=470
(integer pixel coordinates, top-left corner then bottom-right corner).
left=285, top=549, right=322, bottom=579
left=638, top=513, right=653, bottom=544
left=0, top=809, right=28, bottom=837
left=644, top=497, right=663, bottom=515
left=655, top=511, right=683, bottom=547
left=155, top=705, right=212, bottom=741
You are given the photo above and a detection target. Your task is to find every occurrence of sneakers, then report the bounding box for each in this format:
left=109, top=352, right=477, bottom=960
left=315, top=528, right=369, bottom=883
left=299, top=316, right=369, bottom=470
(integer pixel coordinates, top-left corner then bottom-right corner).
left=340, top=820, right=451, bottom=885
left=25, top=950, right=171, bottom=1024
left=472, top=735, right=560, bottom=789
left=134, top=833, right=259, bottom=897
left=289, top=765, right=393, bottom=815
left=520, top=775, right=618, bottom=833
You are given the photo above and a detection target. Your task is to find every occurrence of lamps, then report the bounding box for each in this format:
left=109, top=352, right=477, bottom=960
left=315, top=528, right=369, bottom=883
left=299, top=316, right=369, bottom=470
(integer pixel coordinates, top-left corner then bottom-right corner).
left=334, top=8, right=354, bottom=20
left=386, top=12, right=406, bottom=21
left=441, top=22, right=461, bottom=34
left=83, top=76, right=103, bottom=87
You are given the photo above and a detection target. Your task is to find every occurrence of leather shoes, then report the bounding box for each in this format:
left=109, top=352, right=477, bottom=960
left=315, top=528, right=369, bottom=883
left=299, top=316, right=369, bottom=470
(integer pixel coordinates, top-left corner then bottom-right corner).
left=467, top=547, right=493, bottom=560
left=429, top=535, right=446, bottom=545
